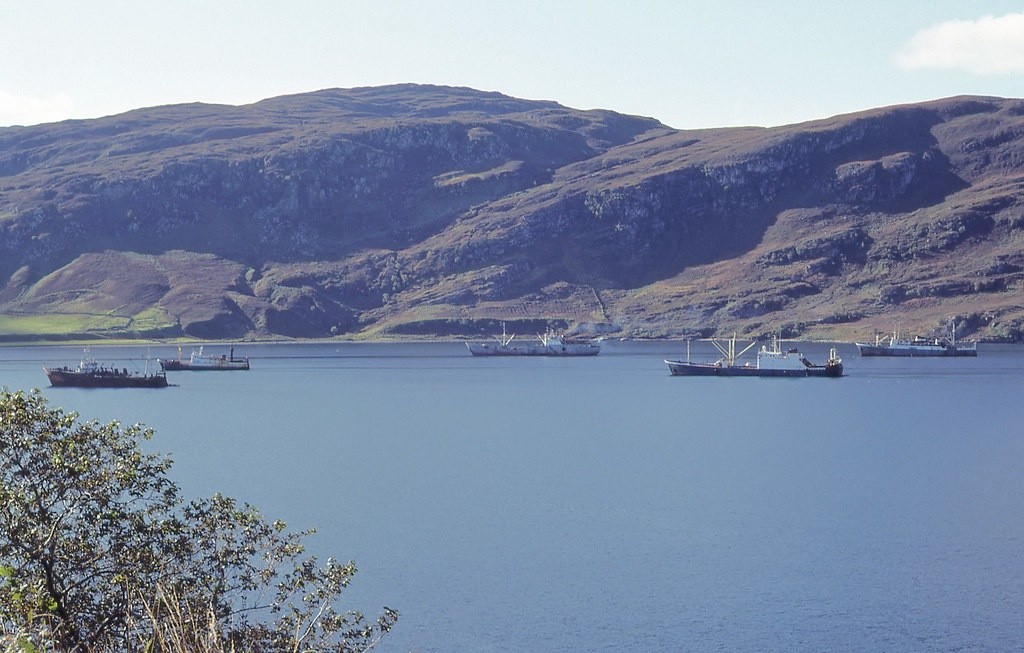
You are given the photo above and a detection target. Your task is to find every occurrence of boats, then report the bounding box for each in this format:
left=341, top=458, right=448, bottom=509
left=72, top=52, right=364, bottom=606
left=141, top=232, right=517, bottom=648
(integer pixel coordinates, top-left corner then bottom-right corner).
left=43, top=344, right=169, bottom=389
left=855, top=320, right=978, bottom=357
left=158, top=339, right=251, bottom=371
left=664, top=329, right=844, bottom=378
left=464, top=321, right=601, bottom=358
